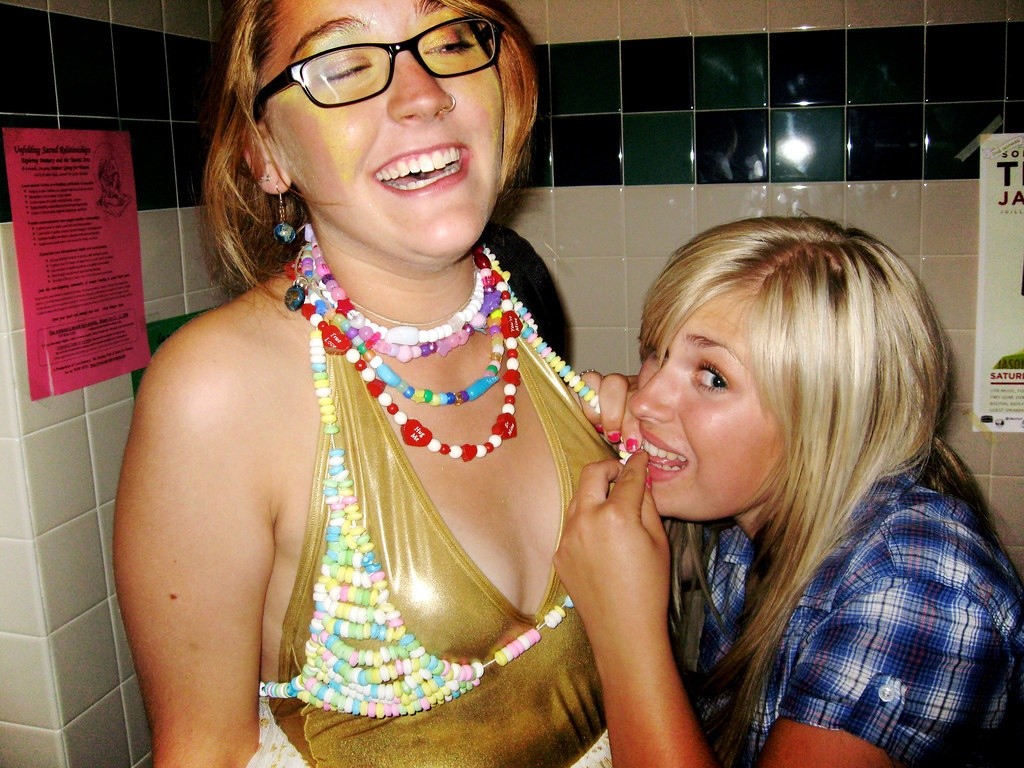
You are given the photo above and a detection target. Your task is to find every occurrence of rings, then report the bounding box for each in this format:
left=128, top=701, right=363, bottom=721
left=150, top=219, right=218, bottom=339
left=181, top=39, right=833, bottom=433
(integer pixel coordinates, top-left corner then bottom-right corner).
left=579, top=369, right=601, bottom=376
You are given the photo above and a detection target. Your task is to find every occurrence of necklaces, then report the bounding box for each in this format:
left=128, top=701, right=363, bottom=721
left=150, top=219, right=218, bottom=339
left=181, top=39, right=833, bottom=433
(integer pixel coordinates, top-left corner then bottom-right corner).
left=279, top=209, right=639, bottom=466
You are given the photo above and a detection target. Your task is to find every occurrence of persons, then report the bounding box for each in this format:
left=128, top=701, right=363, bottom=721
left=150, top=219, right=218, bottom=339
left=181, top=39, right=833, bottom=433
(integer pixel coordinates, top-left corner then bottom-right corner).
left=556, top=217, right=1024, bottom=768
left=111, top=0, right=620, bottom=767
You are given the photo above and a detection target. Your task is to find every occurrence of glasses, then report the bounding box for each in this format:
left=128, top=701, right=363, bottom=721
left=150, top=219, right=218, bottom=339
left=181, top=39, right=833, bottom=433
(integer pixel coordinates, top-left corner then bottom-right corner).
left=252, top=13, right=507, bottom=119
